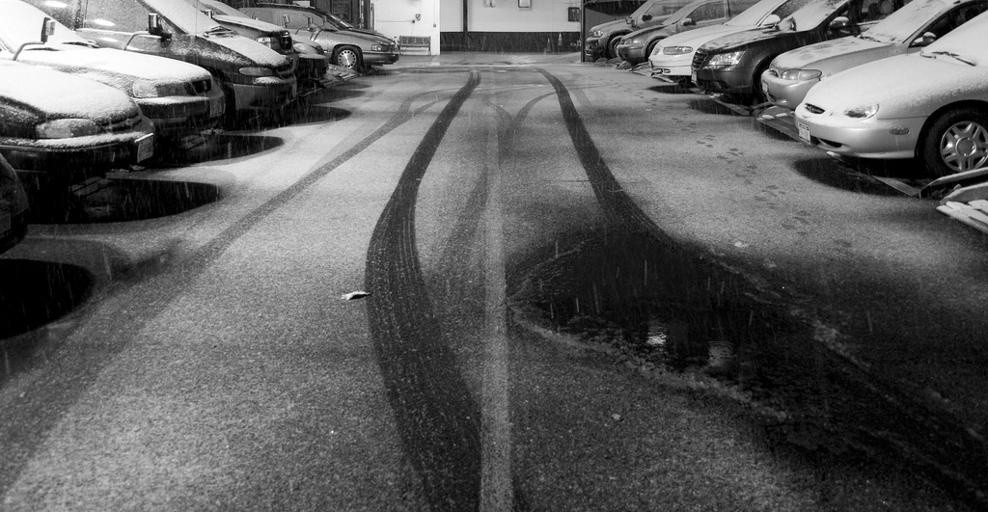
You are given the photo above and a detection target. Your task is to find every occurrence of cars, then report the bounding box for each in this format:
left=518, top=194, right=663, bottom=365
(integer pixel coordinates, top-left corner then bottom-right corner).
left=760, top=0, right=988, bottom=113
left=585, top=0, right=694, bottom=61
left=0, top=57, right=155, bottom=202
left=648, top=0, right=813, bottom=86
left=616, top=0, right=763, bottom=67
left=2, top=1, right=227, bottom=154
left=689, top=0, right=913, bottom=106
left=792, top=8, right=988, bottom=186
left=187, top=0, right=398, bottom=84
left=25, top=0, right=300, bottom=128
left=0, top=153, right=32, bottom=258
